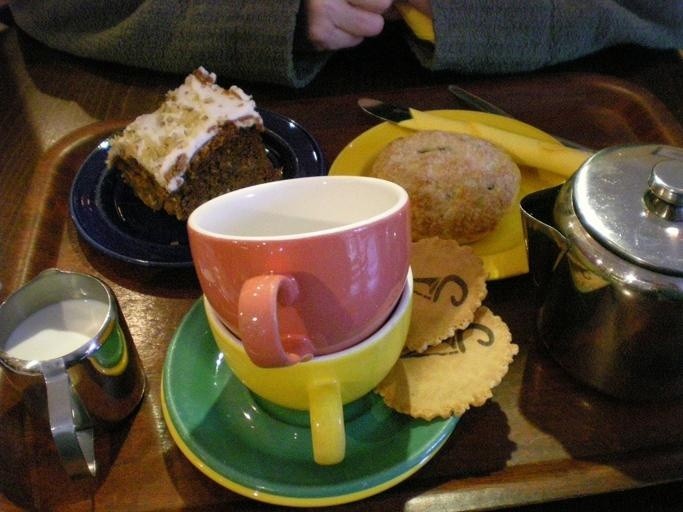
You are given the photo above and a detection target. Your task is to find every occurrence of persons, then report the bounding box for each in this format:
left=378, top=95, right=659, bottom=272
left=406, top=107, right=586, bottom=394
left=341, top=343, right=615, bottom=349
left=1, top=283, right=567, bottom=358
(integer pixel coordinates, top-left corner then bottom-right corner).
left=0, top=0, right=683, bottom=88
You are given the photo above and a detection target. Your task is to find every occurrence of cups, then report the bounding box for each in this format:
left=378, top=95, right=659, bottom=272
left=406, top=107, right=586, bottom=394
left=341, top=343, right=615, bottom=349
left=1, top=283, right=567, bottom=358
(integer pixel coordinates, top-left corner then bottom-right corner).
left=0, top=268, right=148, bottom=480
left=187, top=175, right=413, bottom=368
left=201, top=265, right=413, bottom=466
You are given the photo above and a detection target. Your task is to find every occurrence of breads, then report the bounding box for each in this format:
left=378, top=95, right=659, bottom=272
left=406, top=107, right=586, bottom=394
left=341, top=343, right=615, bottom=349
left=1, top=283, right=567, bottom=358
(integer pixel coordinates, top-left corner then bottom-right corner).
left=369, top=132, right=522, bottom=246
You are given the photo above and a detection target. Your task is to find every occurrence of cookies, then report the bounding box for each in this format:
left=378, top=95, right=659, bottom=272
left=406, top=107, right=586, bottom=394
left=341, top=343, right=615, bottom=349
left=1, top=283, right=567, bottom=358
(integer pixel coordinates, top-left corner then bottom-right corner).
left=400, top=233, right=488, bottom=353
left=372, top=302, right=518, bottom=422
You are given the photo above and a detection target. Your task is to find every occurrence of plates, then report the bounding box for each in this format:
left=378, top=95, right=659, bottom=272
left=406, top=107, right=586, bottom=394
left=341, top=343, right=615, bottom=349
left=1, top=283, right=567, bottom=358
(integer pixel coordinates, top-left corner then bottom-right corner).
left=157, top=295, right=467, bottom=512
left=329, top=109, right=574, bottom=282
left=71, top=114, right=323, bottom=280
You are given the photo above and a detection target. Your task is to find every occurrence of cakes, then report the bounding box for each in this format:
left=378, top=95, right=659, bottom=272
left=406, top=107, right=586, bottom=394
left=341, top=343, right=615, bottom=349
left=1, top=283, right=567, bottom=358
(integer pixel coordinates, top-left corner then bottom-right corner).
left=110, top=62, right=283, bottom=223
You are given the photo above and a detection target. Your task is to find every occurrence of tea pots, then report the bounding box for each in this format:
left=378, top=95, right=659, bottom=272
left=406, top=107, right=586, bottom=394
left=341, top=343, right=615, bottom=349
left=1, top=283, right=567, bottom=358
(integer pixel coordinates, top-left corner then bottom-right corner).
left=523, top=141, right=683, bottom=402
